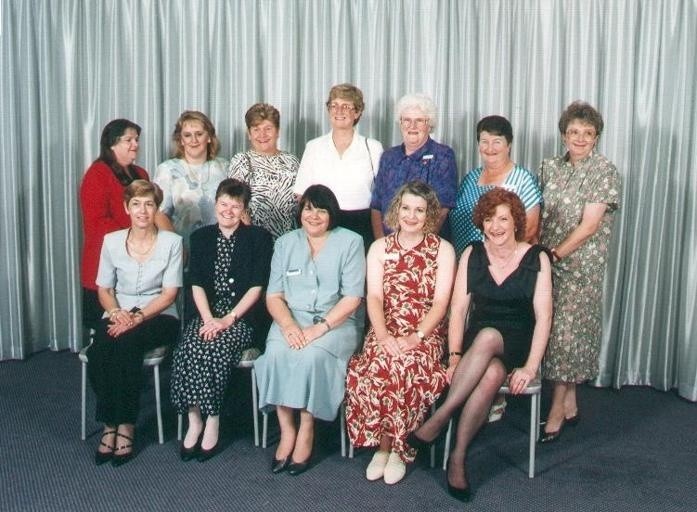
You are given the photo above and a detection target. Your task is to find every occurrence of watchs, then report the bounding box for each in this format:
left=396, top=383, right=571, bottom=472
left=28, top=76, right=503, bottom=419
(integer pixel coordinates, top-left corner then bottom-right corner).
left=414, top=330, right=425, bottom=343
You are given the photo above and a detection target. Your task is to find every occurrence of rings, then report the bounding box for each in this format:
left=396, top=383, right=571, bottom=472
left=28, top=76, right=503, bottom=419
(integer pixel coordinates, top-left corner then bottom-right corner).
left=287, top=334, right=292, bottom=338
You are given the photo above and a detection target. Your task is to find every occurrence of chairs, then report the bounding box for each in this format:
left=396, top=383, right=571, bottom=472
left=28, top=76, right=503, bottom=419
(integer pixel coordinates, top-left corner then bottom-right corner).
left=262, top=402, right=346, bottom=457
left=177, top=347, right=260, bottom=446
left=349, top=401, right=436, bottom=468
left=443, top=362, right=542, bottom=479
left=79, top=285, right=184, bottom=444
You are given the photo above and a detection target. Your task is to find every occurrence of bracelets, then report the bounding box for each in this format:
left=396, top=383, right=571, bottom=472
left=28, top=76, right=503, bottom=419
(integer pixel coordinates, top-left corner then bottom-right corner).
left=448, top=352, right=464, bottom=356
left=135, top=311, right=144, bottom=318
left=550, top=248, right=561, bottom=261
left=109, top=307, right=122, bottom=314
left=229, top=311, right=237, bottom=322
left=320, top=318, right=331, bottom=331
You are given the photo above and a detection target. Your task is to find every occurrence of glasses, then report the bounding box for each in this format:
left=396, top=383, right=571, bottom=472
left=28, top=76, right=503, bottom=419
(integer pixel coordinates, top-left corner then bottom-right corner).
left=569, top=129, right=596, bottom=139
left=327, top=104, right=355, bottom=114
left=399, top=117, right=428, bottom=127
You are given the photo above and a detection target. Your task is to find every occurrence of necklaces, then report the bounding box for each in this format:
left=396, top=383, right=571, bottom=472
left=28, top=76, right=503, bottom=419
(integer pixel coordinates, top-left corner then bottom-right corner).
left=130, top=229, right=156, bottom=255
left=185, top=158, right=209, bottom=183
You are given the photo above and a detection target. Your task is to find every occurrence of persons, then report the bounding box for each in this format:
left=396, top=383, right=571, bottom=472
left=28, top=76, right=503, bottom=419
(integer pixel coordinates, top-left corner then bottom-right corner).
left=447, top=116, right=542, bottom=424
left=408, top=189, right=553, bottom=501
left=537, top=103, right=621, bottom=444
left=153, top=111, right=229, bottom=322
left=369, top=94, right=456, bottom=240
left=345, top=182, right=456, bottom=484
left=293, top=84, right=384, bottom=258
left=87, top=180, right=184, bottom=468
left=253, top=185, right=365, bottom=475
left=81, top=119, right=150, bottom=348
left=169, top=179, right=273, bottom=463
left=229, top=104, right=300, bottom=244
